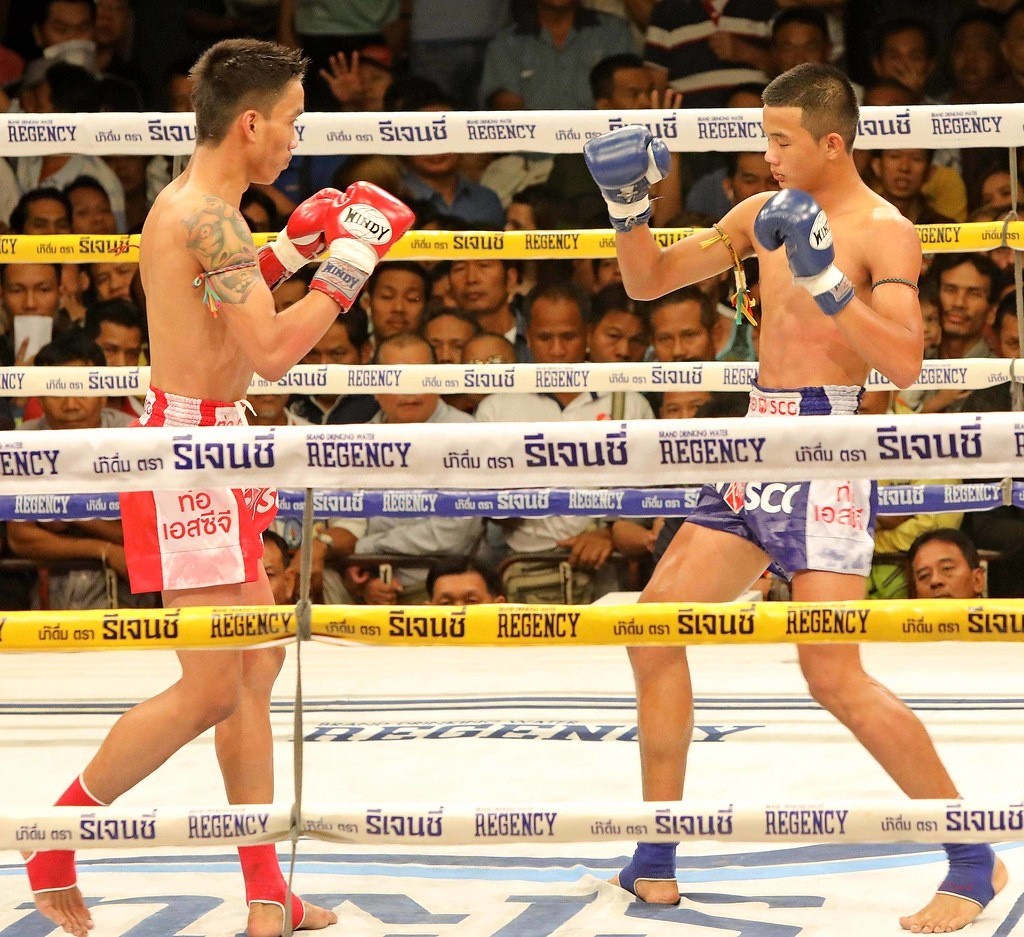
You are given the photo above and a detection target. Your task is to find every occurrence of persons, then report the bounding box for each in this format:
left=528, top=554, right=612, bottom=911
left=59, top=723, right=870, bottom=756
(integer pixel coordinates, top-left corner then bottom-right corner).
left=19, top=38, right=415, bottom=937
left=0, top=0, right=1024, bottom=612
left=581, top=61, right=1009, bottom=933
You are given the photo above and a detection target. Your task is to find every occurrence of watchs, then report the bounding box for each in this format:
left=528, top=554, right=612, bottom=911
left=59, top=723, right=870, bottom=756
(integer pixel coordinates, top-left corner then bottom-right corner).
left=317, top=533, right=334, bottom=549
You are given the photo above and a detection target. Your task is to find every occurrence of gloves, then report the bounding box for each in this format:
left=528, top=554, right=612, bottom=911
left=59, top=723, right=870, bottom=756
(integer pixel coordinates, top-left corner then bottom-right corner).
left=257, top=187, right=344, bottom=291
left=310, top=180, right=416, bottom=315
left=583, top=124, right=672, bottom=233
left=753, top=190, right=856, bottom=316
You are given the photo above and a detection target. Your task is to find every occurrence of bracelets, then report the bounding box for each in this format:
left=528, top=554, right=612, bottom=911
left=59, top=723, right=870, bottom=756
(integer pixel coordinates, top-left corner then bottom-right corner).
left=102, top=543, right=111, bottom=571
